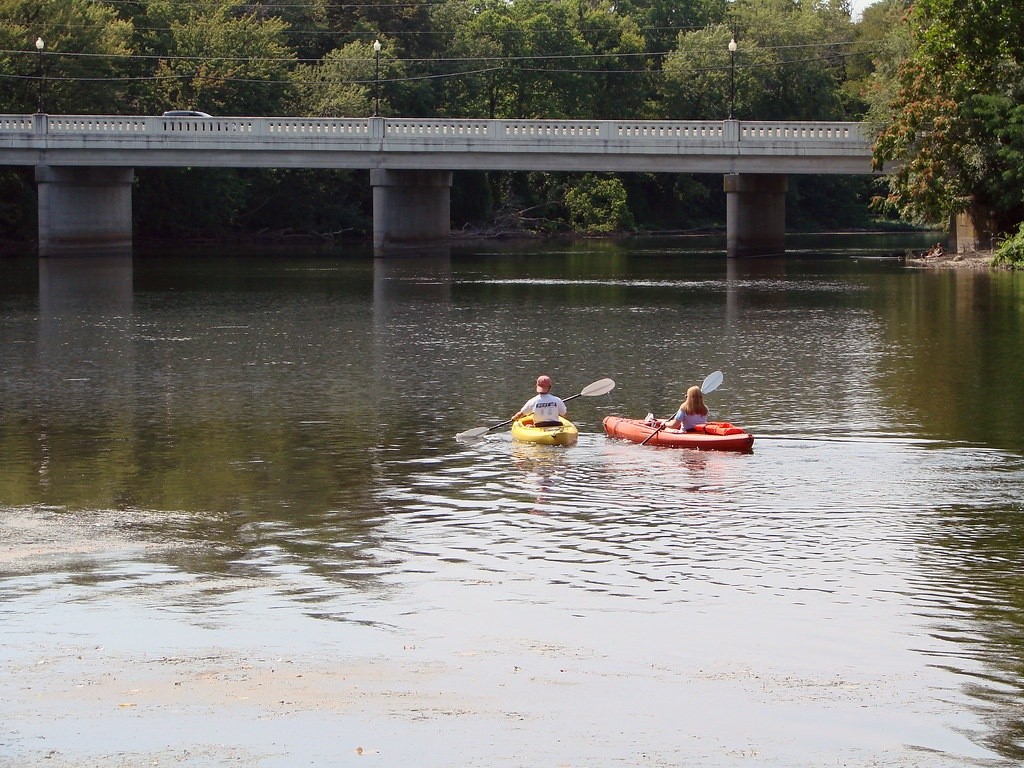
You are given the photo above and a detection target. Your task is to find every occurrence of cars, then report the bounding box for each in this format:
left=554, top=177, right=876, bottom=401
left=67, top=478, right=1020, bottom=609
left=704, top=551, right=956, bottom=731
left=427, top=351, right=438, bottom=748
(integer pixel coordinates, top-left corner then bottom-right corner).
left=163, top=110, right=227, bottom=131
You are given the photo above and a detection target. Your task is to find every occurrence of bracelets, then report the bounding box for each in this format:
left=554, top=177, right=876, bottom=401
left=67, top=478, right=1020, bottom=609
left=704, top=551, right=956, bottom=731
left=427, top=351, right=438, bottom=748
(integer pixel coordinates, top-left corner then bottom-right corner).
left=664, top=422, right=666, bottom=426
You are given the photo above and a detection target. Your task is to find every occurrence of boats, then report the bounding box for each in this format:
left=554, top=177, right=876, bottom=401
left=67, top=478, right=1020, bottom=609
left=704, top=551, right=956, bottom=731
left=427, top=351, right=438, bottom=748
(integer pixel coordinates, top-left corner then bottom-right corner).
left=511, top=412, right=578, bottom=446
left=603, top=414, right=754, bottom=452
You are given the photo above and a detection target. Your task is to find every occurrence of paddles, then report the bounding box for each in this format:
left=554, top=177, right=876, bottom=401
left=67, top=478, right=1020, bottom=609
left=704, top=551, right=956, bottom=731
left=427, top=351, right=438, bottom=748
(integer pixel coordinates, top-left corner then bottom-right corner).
left=641, top=371, right=724, bottom=445
left=458, top=378, right=616, bottom=438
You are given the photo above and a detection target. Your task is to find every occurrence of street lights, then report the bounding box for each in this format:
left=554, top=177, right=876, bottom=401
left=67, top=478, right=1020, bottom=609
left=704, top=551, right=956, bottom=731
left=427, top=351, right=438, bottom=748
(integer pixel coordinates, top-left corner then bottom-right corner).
left=373, top=39, right=382, bottom=117
left=35, top=36, right=46, bottom=113
left=727, top=36, right=737, bottom=120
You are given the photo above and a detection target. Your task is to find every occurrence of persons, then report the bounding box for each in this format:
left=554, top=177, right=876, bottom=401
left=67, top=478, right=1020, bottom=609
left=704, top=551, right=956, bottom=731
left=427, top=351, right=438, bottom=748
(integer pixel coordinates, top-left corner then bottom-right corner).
left=512, top=376, right=566, bottom=427
left=660, top=386, right=708, bottom=433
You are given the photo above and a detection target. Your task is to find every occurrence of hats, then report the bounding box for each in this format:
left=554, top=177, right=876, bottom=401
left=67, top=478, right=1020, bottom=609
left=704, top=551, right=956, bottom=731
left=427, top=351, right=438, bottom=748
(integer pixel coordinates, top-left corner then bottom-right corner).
left=537, top=375, right=551, bottom=393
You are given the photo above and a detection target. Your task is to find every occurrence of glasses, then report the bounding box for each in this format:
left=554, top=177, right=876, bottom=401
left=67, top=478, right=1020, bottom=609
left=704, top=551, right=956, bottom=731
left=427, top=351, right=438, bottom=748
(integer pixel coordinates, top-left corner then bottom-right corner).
left=685, top=395, right=688, bottom=398
